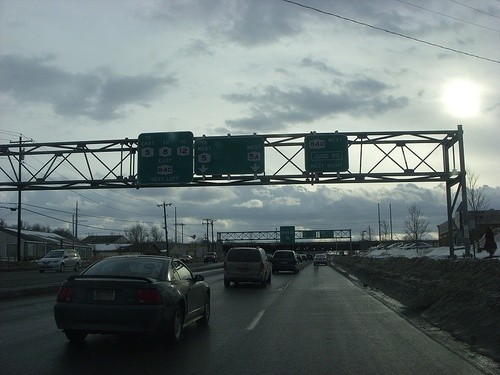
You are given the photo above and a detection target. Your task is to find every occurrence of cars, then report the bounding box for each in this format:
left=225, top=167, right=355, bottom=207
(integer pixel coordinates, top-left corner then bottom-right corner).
left=179, top=253, right=194, bottom=264
left=53, top=251, right=212, bottom=343
left=313, top=254, right=327, bottom=266
left=264, top=248, right=361, bottom=263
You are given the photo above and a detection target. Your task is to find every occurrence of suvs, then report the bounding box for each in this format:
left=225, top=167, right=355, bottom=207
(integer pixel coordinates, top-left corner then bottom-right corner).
left=36, top=249, right=81, bottom=274
left=271, top=249, right=300, bottom=274
left=202, top=252, right=218, bottom=262
left=222, top=245, right=273, bottom=289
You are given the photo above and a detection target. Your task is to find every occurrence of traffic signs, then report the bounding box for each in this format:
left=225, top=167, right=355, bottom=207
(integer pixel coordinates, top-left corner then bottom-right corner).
left=138, top=132, right=194, bottom=184
left=279, top=225, right=295, bottom=243
left=304, top=131, right=349, bottom=172
left=303, top=230, right=317, bottom=240
left=194, top=135, right=266, bottom=175
left=320, top=230, right=333, bottom=238
